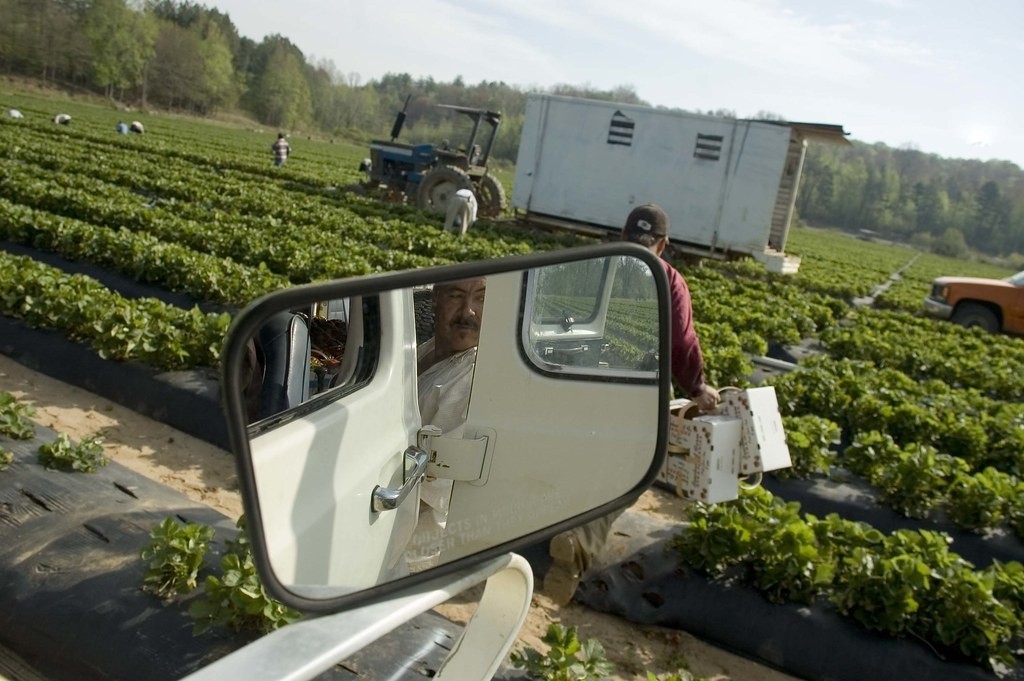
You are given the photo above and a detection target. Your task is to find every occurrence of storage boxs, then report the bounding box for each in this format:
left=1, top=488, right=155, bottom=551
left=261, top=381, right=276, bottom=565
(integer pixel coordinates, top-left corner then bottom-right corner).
left=656, top=404, right=743, bottom=504
left=718, top=385, right=792, bottom=476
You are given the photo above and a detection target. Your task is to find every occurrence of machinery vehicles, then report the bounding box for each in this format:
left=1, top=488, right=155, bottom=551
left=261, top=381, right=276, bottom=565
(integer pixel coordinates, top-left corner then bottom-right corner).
left=357, top=96, right=852, bottom=278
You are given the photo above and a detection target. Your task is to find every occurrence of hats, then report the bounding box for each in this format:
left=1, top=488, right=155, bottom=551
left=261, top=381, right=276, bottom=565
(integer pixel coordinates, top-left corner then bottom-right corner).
left=625, top=204, right=668, bottom=235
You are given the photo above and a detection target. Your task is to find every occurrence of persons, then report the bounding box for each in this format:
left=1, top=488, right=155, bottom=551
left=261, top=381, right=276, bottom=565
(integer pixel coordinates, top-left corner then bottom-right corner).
left=418, top=278, right=486, bottom=515
left=272, top=133, right=292, bottom=168
left=8, top=107, right=23, bottom=119
left=359, top=159, right=371, bottom=172
left=52, top=114, right=71, bottom=125
left=443, top=188, right=478, bottom=242
left=116, top=120, right=144, bottom=135
left=541, top=206, right=722, bottom=607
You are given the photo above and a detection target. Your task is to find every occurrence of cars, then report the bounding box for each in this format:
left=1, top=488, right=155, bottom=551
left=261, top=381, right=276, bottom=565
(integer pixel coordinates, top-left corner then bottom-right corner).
left=924, top=271, right=1024, bottom=338
left=236, top=255, right=663, bottom=599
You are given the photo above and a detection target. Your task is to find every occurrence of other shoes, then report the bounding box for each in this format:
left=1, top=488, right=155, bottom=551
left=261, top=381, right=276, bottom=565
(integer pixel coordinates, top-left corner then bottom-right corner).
left=544, top=531, right=580, bottom=608
